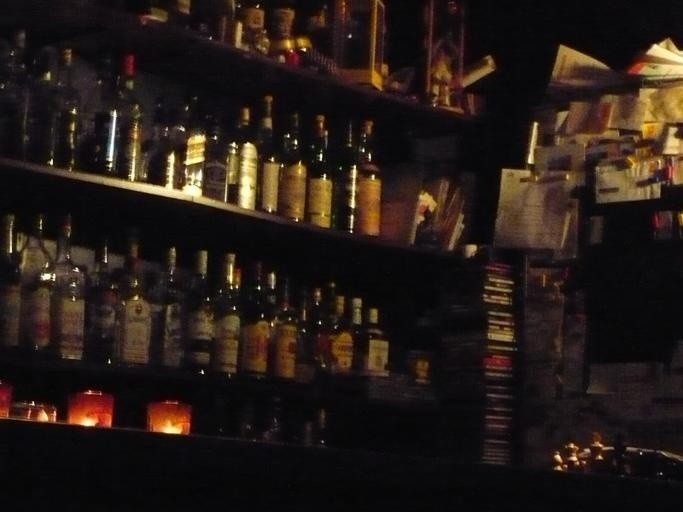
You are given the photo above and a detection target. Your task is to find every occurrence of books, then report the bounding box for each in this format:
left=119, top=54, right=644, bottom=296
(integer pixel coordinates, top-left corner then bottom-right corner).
left=373, top=162, right=469, bottom=253
left=436, top=257, right=518, bottom=467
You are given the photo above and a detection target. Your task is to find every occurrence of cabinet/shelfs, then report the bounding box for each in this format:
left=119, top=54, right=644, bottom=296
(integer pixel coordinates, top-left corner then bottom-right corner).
left=0, top=2, right=483, bottom=473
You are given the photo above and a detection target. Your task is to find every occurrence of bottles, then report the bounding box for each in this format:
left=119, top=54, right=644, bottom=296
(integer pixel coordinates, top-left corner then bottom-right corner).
left=1, top=209, right=438, bottom=456
left=2, top=24, right=382, bottom=239
